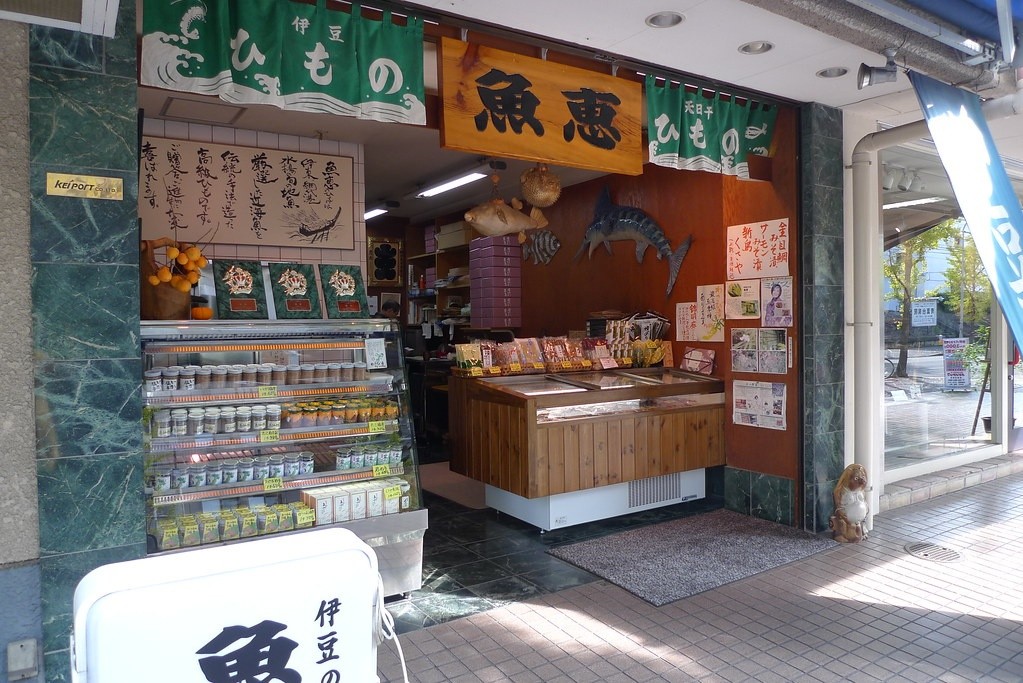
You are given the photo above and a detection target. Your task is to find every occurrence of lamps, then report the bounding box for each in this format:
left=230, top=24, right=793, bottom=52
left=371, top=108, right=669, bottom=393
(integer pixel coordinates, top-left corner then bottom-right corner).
left=883, top=173, right=893, bottom=191
left=364, top=200, right=401, bottom=221
left=856, top=57, right=898, bottom=90
left=897, top=175, right=912, bottom=192
left=910, top=176, right=922, bottom=192
left=402, top=161, right=507, bottom=200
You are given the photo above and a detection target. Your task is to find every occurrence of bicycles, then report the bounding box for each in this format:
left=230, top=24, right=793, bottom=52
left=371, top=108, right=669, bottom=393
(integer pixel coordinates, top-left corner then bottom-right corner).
left=884, top=357, right=895, bottom=380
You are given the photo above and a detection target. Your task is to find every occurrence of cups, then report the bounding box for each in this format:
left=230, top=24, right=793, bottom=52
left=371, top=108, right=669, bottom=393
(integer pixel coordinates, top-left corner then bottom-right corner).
left=145, top=362, right=403, bottom=492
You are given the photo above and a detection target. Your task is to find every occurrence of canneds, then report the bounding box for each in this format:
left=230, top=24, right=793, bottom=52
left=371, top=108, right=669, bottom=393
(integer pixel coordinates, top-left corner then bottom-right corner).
left=144, top=361, right=404, bottom=493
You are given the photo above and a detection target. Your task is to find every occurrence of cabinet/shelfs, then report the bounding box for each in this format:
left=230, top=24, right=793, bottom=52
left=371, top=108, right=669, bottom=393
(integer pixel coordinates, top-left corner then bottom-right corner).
left=404, top=207, right=485, bottom=326
left=139, top=319, right=429, bottom=601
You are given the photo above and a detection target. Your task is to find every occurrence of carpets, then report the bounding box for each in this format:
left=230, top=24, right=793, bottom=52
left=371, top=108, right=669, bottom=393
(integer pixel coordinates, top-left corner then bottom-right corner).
left=543, top=507, right=842, bottom=608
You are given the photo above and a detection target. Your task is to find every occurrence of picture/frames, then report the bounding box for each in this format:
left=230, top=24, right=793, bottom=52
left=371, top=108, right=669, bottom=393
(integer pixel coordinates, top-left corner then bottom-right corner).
left=381, top=292, right=401, bottom=316
left=368, top=235, right=405, bottom=287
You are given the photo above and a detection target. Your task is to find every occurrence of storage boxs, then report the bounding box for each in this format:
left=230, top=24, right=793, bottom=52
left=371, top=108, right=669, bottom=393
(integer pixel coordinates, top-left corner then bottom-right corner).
left=426, top=274, right=436, bottom=281
left=438, top=229, right=479, bottom=250
left=568, top=330, right=587, bottom=339
left=426, top=267, right=436, bottom=276
left=440, top=220, right=471, bottom=234
left=299, top=476, right=401, bottom=527
left=424, top=225, right=437, bottom=254
left=585, top=317, right=625, bottom=338
left=469, top=236, right=522, bottom=328
left=425, top=282, right=435, bottom=289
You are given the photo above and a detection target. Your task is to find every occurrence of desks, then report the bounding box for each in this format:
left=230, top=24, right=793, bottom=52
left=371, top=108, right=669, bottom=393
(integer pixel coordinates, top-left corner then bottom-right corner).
left=403, top=355, right=451, bottom=445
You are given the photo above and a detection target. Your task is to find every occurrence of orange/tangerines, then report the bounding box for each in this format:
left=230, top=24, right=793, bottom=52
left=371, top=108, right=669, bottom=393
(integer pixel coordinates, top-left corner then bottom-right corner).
left=149, top=248, right=208, bottom=293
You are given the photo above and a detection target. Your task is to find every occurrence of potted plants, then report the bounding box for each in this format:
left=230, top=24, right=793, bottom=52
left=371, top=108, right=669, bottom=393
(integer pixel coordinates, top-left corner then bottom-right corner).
left=957, top=324, right=1016, bottom=433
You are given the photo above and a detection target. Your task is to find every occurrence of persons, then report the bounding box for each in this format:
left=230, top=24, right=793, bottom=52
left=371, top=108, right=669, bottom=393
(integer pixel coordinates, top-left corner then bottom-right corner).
left=373, top=299, right=401, bottom=318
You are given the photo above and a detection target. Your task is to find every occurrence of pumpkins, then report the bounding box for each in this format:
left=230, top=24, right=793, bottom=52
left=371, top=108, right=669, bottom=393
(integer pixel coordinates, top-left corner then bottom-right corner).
left=191, top=307, right=213, bottom=320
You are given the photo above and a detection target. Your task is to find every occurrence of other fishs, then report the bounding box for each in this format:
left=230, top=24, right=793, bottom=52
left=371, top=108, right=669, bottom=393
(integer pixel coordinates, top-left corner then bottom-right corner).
left=523, top=229, right=561, bottom=265
left=464, top=198, right=548, bottom=239
